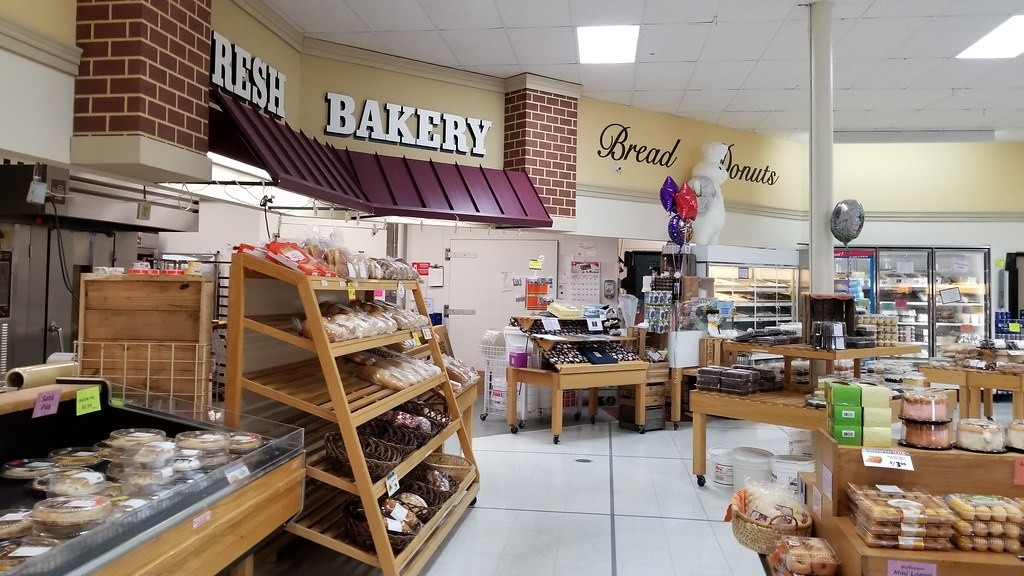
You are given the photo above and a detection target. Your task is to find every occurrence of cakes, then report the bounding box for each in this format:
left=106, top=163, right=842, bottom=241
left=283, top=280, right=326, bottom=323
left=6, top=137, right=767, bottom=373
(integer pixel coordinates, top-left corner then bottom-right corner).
left=900, top=386, right=1024, bottom=455
left=0, top=432, right=263, bottom=576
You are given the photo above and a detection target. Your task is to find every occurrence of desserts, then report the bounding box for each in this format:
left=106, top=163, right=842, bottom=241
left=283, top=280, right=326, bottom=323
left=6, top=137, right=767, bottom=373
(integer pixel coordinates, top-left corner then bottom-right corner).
left=858, top=319, right=898, bottom=348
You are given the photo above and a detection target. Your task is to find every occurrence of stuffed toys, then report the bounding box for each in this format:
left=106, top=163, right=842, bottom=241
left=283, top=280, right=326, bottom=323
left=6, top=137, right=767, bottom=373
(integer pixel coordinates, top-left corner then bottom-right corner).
left=601, top=304, right=622, bottom=336
left=707, top=313, right=724, bottom=338
left=686, top=141, right=729, bottom=245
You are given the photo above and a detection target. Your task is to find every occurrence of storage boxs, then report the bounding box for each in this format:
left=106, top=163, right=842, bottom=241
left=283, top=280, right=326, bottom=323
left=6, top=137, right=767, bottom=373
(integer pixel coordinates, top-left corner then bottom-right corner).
left=824, top=379, right=892, bottom=447
left=994, top=349, right=1024, bottom=363
left=618, top=361, right=668, bottom=431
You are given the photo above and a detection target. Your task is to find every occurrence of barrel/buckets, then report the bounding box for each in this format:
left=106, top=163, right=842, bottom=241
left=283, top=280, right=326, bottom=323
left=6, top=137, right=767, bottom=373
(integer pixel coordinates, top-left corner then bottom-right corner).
left=706, top=446, right=733, bottom=493
left=731, top=446, right=774, bottom=492
left=504, top=326, right=532, bottom=361
left=771, top=454, right=817, bottom=495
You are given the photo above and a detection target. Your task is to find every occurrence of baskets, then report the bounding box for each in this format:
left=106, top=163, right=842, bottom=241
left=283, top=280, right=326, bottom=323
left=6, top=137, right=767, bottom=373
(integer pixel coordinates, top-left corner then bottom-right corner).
left=730, top=487, right=813, bottom=555
left=324, top=400, right=452, bottom=481
left=342, top=453, right=474, bottom=555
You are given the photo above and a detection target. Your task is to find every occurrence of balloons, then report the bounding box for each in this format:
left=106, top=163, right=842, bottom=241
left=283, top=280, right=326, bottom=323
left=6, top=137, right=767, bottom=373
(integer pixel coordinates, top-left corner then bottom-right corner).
left=675, top=182, right=698, bottom=221
left=668, top=214, right=694, bottom=245
left=830, top=199, right=865, bottom=245
left=659, top=176, right=680, bottom=214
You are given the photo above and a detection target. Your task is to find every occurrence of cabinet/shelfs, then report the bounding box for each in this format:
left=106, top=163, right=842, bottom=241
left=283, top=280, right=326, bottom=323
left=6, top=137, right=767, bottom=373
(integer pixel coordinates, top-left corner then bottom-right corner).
left=759, top=427, right=1024, bottom=576
left=688, top=340, right=921, bottom=487
left=223, top=252, right=481, bottom=576
left=689, top=245, right=800, bottom=331
left=832, top=245, right=992, bottom=359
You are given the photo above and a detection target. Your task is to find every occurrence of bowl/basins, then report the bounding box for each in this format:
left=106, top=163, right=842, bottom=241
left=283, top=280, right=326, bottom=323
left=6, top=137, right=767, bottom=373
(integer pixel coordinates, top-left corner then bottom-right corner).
left=694, top=364, right=785, bottom=397
left=0, top=427, right=277, bottom=576
left=809, top=321, right=878, bottom=350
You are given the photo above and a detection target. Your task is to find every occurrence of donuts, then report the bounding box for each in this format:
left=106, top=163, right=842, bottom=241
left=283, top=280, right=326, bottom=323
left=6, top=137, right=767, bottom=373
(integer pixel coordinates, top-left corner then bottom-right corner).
left=783, top=538, right=838, bottom=576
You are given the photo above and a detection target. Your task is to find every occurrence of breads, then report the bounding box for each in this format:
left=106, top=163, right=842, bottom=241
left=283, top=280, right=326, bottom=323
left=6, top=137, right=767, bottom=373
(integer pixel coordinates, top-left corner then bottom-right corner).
left=846, top=483, right=1024, bottom=555
left=303, top=244, right=420, bottom=280
left=352, top=470, right=452, bottom=543
left=357, top=355, right=439, bottom=390
left=296, top=299, right=429, bottom=342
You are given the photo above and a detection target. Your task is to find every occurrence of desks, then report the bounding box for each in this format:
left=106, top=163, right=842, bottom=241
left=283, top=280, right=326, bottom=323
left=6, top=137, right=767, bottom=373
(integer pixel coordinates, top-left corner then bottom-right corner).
left=505, top=365, right=647, bottom=445
left=919, top=364, right=1024, bottom=419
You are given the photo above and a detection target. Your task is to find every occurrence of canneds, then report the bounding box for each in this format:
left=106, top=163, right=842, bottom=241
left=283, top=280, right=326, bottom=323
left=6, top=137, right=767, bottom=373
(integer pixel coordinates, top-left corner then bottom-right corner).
left=995, top=312, right=1024, bottom=340
